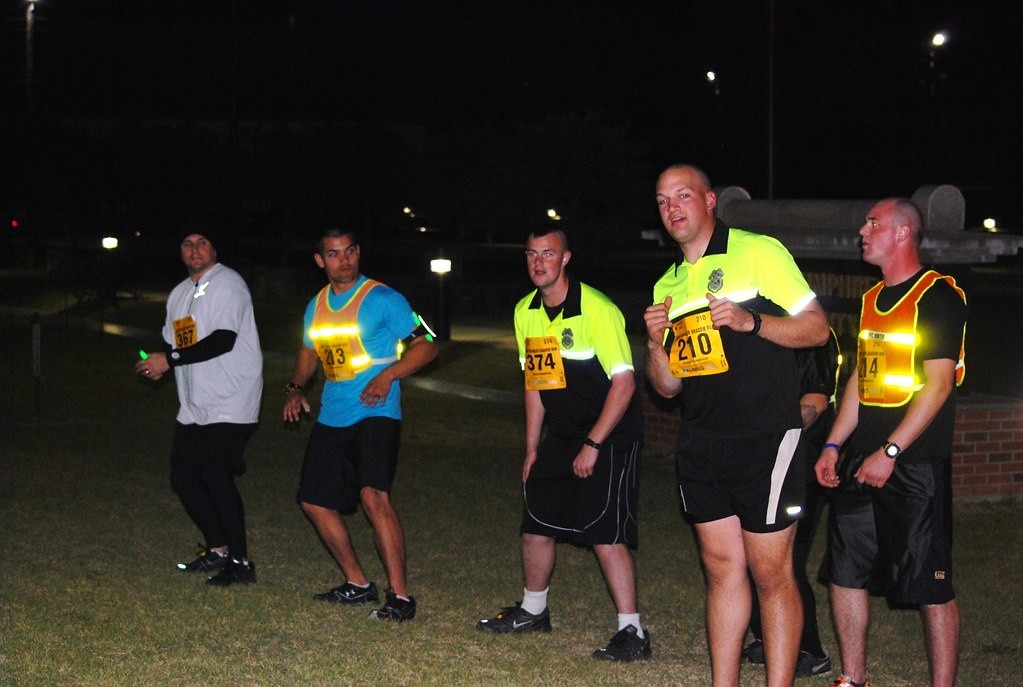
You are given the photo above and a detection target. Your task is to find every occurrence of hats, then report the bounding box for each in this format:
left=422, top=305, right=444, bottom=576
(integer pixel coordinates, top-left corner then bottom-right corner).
left=175, top=218, right=216, bottom=247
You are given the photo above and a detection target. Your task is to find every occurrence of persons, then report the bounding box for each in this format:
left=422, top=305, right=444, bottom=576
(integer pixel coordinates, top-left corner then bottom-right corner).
left=136, top=229, right=264, bottom=587
left=284, top=226, right=439, bottom=625
left=814, top=198, right=969, bottom=687
left=741, top=325, right=832, bottom=679
left=643, top=163, right=830, bottom=687
left=475, top=224, right=652, bottom=663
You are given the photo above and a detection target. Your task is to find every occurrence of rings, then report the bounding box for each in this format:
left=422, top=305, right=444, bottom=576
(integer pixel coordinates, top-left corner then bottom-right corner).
left=376, top=394, right=380, bottom=398
left=146, top=370, right=151, bottom=375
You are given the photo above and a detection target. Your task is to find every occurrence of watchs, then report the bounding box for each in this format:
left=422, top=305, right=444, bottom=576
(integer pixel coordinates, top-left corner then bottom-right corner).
left=882, top=440, right=901, bottom=459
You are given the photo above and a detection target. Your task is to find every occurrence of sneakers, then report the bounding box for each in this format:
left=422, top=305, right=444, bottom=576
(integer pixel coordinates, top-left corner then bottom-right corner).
left=312, top=580, right=379, bottom=605
left=591, top=624, right=651, bottom=661
left=740, top=639, right=766, bottom=666
left=793, top=650, right=832, bottom=678
left=206, top=556, right=255, bottom=584
left=477, top=601, right=552, bottom=634
left=369, top=591, right=415, bottom=622
left=176, top=543, right=229, bottom=571
left=832, top=675, right=870, bottom=687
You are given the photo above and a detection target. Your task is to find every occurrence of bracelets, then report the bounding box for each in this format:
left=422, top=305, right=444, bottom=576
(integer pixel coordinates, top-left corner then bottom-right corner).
left=822, top=444, right=841, bottom=450
left=286, top=383, right=301, bottom=396
left=584, top=437, right=600, bottom=449
left=746, top=309, right=761, bottom=335
